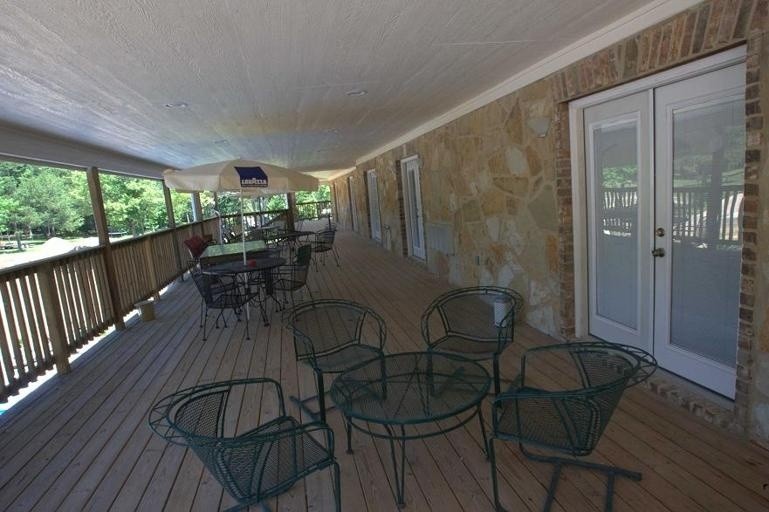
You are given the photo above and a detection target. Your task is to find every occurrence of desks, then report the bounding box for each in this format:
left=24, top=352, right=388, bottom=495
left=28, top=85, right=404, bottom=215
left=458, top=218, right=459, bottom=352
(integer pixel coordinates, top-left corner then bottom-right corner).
left=329, top=351, right=492, bottom=510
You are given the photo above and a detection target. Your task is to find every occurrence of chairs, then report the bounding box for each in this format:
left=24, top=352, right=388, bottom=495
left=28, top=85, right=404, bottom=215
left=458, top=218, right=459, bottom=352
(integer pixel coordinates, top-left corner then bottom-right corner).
left=184, top=223, right=338, bottom=341
left=420, top=286, right=527, bottom=409
left=280, top=300, right=388, bottom=427
left=488, top=341, right=657, bottom=512
left=150, top=378, right=342, bottom=512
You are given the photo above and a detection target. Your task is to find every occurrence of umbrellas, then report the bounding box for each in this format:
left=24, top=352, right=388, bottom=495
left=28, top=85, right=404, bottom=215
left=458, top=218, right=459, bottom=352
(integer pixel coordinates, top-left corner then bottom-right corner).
left=162, top=158, right=319, bottom=322
